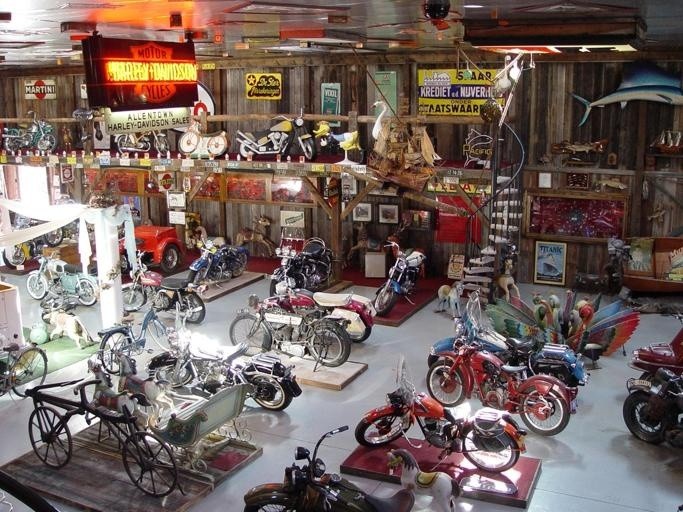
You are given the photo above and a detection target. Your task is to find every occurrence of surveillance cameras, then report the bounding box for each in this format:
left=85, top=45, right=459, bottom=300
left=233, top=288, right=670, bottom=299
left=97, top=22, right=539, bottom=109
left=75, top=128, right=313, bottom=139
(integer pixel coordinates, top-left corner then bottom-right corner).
left=427, top=0, right=451, bottom=19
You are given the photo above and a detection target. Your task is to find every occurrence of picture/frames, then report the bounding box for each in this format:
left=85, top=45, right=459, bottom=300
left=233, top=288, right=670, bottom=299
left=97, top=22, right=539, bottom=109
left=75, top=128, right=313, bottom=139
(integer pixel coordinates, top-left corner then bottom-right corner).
left=523, top=188, right=632, bottom=245
left=534, top=241, right=565, bottom=286
left=341, top=177, right=400, bottom=225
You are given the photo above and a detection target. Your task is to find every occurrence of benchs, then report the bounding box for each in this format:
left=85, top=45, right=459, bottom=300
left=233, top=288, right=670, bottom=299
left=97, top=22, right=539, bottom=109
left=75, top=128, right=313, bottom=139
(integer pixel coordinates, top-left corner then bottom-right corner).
left=148, top=382, right=249, bottom=446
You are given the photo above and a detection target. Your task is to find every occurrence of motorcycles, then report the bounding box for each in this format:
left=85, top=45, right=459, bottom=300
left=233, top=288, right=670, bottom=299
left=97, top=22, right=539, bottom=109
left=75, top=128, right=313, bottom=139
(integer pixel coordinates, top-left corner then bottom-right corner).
left=1, top=111, right=56, bottom=155
left=117, top=128, right=171, bottom=157
left=315, top=121, right=365, bottom=163
left=236, top=106, right=316, bottom=163
left=178, top=118, right=228, bottom=158
